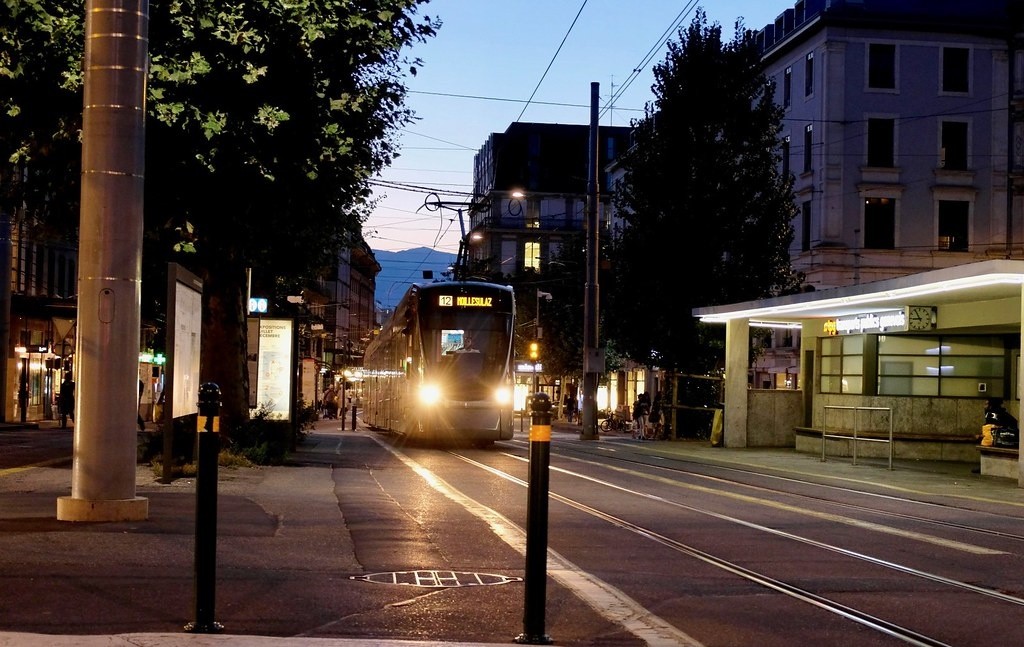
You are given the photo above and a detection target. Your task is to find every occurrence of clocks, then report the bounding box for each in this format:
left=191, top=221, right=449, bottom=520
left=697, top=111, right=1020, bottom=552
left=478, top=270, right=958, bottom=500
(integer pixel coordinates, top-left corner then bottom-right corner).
left=905, top=305, right=938, bottom=332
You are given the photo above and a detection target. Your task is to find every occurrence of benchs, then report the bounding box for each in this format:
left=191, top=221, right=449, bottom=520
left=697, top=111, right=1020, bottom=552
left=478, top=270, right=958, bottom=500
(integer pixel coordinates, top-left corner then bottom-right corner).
left=790, top=425, right=1021, bottom=480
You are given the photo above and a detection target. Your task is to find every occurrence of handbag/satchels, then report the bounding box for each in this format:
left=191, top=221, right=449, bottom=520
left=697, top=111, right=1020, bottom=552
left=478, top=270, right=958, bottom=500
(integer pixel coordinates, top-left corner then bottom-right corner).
left=648, top=411, right=661, bottom=423
left=632, top=405, right=643, bottom=420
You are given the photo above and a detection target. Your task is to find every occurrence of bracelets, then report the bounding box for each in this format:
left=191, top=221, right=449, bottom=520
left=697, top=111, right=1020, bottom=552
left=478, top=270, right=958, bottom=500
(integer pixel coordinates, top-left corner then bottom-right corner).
left=979, top=435, right=981, bottom=439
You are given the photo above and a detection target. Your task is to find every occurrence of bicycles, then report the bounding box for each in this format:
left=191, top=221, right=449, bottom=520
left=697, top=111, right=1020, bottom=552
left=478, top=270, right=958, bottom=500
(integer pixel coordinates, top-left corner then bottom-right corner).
left=601, top=411, right=627, bottom=434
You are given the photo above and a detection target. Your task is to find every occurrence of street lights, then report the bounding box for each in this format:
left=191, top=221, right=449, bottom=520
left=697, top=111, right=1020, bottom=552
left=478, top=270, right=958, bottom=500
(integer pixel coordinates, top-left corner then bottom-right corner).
left=532, top=289, right=553, bottom=398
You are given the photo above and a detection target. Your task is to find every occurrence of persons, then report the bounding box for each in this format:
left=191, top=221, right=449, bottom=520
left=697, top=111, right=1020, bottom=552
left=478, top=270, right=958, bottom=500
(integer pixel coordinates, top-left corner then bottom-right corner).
left=59, top=373, right=75, bottom=429
left=555, top=390, right=661, bottom=441
left=139, top=379, right=146, bottom=431
left=970, top=397, right=1018, bottom=473
left=322, top=383, right=353, bottom=420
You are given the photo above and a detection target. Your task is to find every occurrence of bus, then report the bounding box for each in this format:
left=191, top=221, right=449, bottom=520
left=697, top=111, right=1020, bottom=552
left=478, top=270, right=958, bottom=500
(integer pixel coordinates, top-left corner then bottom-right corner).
left=360, top=204, right=516, bottom=443
